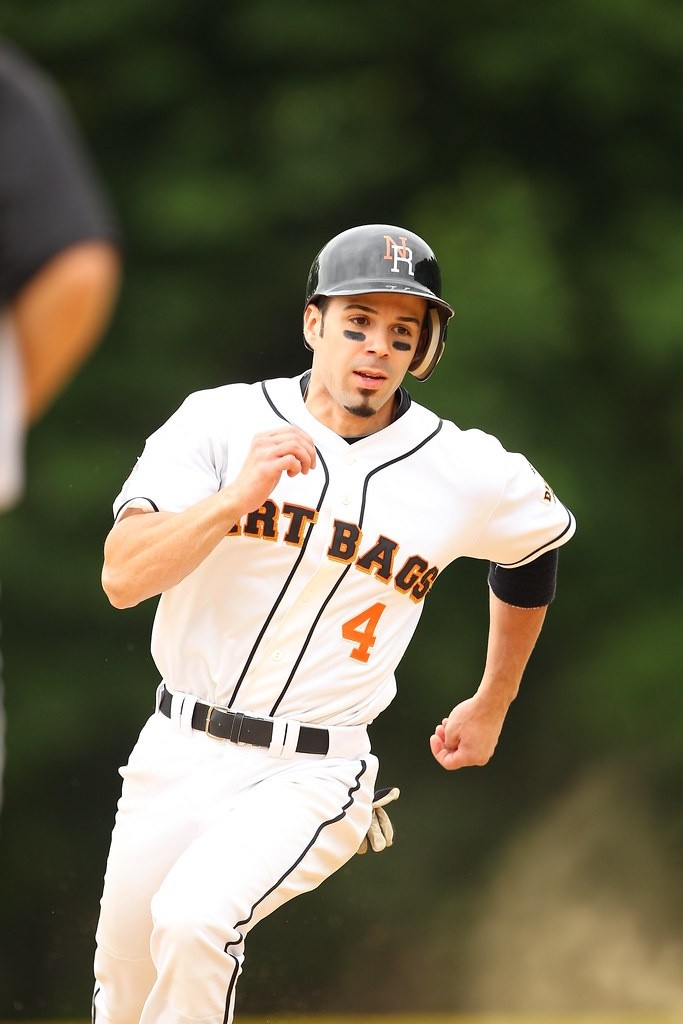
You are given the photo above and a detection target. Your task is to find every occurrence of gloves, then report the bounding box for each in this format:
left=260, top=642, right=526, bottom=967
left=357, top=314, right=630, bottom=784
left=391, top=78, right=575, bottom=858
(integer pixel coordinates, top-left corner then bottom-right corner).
left=356, top=787, right=402, bottom=855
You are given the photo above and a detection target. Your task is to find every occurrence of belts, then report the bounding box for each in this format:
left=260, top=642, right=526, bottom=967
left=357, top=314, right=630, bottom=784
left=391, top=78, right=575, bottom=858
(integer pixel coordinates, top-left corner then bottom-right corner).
left=158, top=683, right=329, bottom=755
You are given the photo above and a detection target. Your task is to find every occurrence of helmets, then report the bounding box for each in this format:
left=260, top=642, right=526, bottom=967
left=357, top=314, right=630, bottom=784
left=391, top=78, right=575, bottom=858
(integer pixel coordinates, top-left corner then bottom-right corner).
left=301, top=224, right=456, bottom=353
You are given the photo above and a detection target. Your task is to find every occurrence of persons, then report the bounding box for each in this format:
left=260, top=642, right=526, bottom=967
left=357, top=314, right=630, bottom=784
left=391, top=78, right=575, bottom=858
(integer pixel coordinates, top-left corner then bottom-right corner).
left=0, top=48, right=125, bottom=512
left=89, top=224, right=576, bottom=1023
